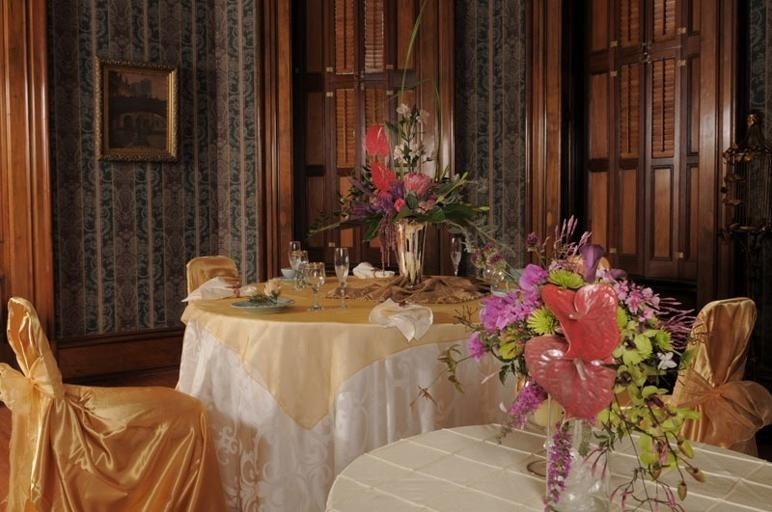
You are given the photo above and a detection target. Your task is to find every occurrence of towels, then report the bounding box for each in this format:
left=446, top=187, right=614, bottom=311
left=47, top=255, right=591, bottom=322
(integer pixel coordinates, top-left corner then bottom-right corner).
left=366, top=296, right=436, bottom=343
left=351, top=262, right=395, bottom=283
left=177, top=274, right=260, bottom=304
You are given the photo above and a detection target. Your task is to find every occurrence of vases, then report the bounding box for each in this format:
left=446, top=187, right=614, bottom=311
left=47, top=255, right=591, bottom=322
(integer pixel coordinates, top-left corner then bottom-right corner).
left=388, top=216, right=433, bottom=287
left=538, top=388, right=612, bottom=512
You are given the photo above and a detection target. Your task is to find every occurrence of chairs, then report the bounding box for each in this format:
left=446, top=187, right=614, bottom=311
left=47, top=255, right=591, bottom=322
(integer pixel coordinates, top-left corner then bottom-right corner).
left=639, top=293, right=772, bottom=462
left=0, top=293, right=228, bottom=512
left=185, top=251, right=242, bottom=303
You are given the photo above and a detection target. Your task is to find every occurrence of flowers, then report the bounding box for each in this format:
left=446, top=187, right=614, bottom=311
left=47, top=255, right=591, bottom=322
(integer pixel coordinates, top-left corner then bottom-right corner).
left=296, top=0, right=528, bottom=261
left=247, top=276, right=285, bottom=306
left=405, top=210, right=723, bottom=512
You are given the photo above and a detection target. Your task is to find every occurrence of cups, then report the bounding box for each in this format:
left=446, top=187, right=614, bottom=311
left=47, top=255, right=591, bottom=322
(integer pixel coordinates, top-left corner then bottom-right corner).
left=472, top=244, right=512, bottom=299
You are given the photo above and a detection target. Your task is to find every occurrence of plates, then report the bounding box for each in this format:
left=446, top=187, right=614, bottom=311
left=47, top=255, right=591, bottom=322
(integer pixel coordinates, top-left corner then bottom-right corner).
left=233, top=296, right=294, bottom=314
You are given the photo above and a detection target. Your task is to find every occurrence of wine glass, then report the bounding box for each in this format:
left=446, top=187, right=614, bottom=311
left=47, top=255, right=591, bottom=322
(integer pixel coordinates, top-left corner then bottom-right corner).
left=449, top=237, right=461, bottom=275
left=304, top=262, right=327, bottom=313
left=333, top=248, right=352, bottom=311
left=288, top=240, right=309, bottom=297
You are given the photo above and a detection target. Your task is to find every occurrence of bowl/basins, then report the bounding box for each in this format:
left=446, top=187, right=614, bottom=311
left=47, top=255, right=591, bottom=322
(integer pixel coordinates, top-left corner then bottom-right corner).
left=279, top=267, right=296, bottom=279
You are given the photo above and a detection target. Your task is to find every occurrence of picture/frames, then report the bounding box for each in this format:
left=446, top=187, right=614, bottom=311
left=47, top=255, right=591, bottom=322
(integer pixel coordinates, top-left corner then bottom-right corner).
left=87, top=51, right=186, bottom=168
